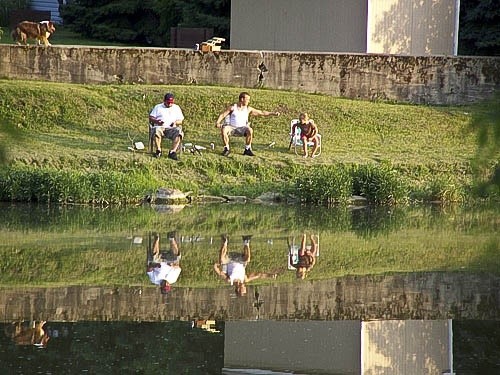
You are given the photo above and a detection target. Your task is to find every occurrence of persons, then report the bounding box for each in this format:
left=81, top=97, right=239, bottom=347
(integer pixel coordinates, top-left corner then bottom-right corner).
left=146, top=231, right=181, bottom=294
left=149, top=93, right=185, bottom=160
left=216, top=92, right=280, bottom=157
left=292, top=113, right=318, bottom=158
left=213, top=233, right=277, bottom=298
left=296, top=234, right=316, bottom=279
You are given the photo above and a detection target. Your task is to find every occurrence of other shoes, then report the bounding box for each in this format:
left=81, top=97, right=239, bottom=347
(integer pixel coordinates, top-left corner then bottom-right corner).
left=242, top=235, right=253, bottom=241
left=223, top=146, right=230, bottom=156
left=167, top=231, right=176, bottom=238
left=169, top=151, right=178, bottom=160
left=244, top=148, right=256, bottom=156
left=152, top=232, right=160, bottom=240
left=222, top=236, right=229, bottom=241
left=155, top=149, right=162, bottom=159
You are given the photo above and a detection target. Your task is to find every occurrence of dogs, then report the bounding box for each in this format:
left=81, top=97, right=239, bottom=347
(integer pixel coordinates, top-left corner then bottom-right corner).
left=10, top=20, right=56, bottom=46
left=8, top=321, right=50, bottom=348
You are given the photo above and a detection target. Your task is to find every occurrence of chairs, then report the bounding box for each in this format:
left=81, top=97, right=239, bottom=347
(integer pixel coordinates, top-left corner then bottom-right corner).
left=287, top=118, right=321, bottom=157
left=224, top=115, right=254, bottom=137
left=147, top=232, right=173, bottom=267
left=288, top=233, right=320, bottom=271
left=148, top=111, right=181, bottom=156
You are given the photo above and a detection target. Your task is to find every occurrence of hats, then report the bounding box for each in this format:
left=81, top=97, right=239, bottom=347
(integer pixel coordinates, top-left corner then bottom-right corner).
left=164, top=93, right=174, bottom=101
